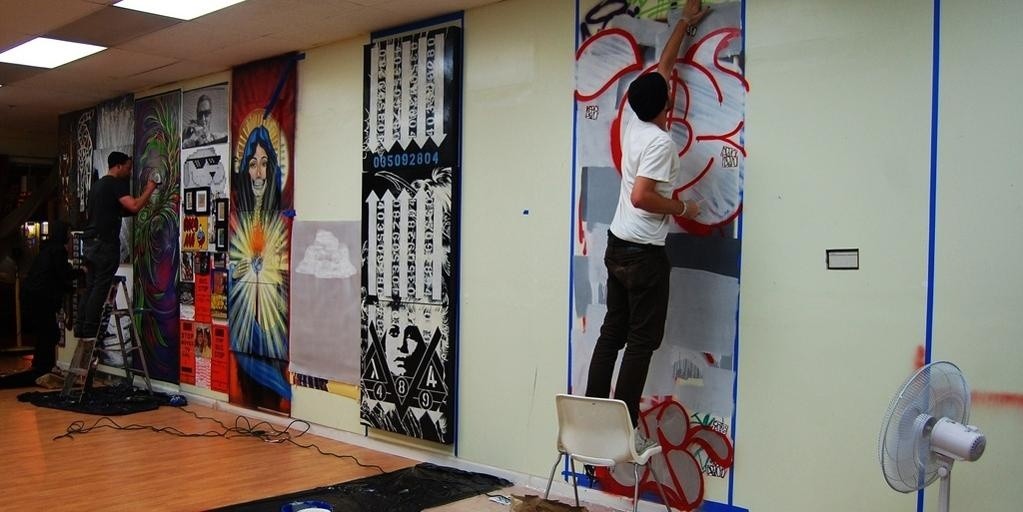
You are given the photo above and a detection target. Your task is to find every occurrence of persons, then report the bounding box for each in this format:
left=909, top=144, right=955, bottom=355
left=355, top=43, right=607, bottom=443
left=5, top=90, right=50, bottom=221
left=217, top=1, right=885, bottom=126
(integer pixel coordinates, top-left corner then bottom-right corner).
left=0, top=220, right=86, bottom=390
left=72, top=151, right=157, bottom=339
left=384, top=323, right=424, bottom=376
left=584, top=1, right=713, bottom=454
left=230, top=126, right=290, bottom=413
left=182, top=95, right=228, bottom=147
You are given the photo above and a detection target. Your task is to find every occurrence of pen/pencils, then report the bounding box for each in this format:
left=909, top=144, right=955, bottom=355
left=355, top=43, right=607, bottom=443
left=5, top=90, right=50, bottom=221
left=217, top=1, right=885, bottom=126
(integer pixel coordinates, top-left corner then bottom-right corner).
left=697, top=197, right=708, bottom=205
left=148, top=178, right=158, bottom=189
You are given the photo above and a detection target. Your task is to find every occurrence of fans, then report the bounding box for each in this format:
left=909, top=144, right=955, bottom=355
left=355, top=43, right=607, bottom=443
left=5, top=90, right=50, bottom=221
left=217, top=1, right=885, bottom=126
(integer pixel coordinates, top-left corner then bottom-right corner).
left=877, top=361, right=987, bottom=512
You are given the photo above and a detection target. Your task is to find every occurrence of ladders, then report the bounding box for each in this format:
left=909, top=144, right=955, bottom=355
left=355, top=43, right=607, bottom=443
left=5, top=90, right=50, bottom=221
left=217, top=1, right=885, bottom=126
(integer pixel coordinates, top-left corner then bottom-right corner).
left=59, top=275, right=153, bottom=404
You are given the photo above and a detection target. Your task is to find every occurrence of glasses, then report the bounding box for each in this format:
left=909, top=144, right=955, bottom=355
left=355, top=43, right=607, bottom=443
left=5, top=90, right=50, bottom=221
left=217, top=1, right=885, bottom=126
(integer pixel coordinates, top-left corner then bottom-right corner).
left=198, top=110, right=211, bottom=121
left=185, top=155, right=221, bottom=169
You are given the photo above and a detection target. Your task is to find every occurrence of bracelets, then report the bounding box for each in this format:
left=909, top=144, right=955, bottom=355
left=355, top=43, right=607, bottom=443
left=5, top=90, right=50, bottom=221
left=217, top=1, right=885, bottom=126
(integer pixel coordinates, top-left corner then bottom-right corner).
left=675, top=200, right=687, bottom=217
left=678, top=16, right=691, bottom=25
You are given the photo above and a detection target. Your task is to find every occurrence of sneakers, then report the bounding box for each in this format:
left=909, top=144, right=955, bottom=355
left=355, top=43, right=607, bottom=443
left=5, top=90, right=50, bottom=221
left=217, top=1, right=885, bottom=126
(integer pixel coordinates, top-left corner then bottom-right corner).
left=633, top=428, right=658, bottom=455
left=74, top=325, right=117, bottom=342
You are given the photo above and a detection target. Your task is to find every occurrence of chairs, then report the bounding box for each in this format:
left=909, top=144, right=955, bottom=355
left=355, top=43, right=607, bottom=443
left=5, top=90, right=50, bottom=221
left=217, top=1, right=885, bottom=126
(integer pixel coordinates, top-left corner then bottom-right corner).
left=543, top=393, right=673, bottom=512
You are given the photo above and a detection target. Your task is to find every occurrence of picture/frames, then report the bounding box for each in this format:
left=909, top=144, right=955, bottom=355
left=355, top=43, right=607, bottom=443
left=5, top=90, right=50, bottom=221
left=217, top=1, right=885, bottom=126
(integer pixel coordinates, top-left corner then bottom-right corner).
left=216, top=198, right=228, bottom=225
left=215, top=225, right=228, bottom=251
left=194, top=186, right=210, bottom=216
left=180, top=250, right=196, bottom=283
left=184, top=188, right=194, bottom=214
